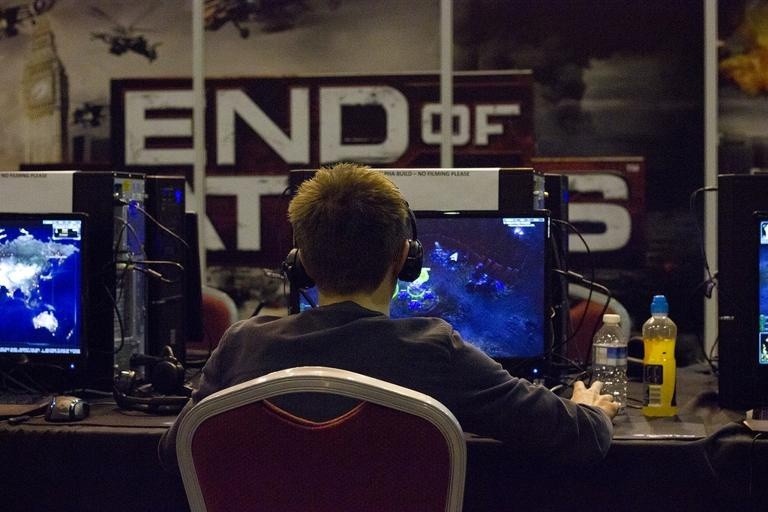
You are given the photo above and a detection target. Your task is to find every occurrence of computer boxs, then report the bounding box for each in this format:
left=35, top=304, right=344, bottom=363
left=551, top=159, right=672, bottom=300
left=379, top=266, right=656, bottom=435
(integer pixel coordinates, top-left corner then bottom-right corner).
left=287, top=168, right=547, bottom=209
left=717, top=173, right=768, bottom=411
left=543, top=174, right=570, bottom=368
left=0, top=171, right=148, bottom=399
left=145, top=175, right=185, bottom=366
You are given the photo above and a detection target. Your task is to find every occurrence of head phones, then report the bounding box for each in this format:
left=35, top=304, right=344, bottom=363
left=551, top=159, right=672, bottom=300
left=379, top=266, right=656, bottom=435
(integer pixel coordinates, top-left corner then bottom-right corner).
left=113, top=352, right=192, bottom=413
left=282, top=176, right=424, bottom=292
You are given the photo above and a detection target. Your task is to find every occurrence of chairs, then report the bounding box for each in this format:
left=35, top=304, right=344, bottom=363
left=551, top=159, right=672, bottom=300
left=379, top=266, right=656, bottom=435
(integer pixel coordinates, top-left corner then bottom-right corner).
left=564, top=284, right=631, bottom=361
left=174, top=366, right=468, bottom=511
left=184, top=288, right=239, bottom=351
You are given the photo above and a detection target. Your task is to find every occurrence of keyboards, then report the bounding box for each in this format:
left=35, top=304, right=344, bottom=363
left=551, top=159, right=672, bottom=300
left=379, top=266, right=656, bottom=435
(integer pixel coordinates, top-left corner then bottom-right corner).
left=0, top=392, right=54, bottom=419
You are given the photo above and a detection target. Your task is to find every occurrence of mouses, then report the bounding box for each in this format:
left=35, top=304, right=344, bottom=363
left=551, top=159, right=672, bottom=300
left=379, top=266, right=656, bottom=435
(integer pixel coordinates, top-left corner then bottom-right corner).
left=44, top=396, right=90, bottom=422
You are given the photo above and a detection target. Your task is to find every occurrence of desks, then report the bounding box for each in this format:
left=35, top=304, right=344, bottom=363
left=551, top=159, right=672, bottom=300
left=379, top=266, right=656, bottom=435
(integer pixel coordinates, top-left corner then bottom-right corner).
left=0, top=349, right=768, bottom=447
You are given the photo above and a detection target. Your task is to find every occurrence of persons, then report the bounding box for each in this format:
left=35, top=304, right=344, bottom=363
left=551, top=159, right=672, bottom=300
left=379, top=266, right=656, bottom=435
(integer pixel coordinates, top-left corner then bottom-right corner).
left=157, top=162, right=623, bottom=511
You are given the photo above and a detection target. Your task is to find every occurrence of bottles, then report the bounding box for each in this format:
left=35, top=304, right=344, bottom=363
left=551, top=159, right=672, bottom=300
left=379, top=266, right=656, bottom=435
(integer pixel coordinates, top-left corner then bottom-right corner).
left=642, top=294, right=677, bottom=417
left=590, top=312, right=628, bottom=414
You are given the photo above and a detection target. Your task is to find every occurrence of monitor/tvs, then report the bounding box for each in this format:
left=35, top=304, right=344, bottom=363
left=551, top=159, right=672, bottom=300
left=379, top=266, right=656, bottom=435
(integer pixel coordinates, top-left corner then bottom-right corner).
left=0, top=212, right=90, bottom=365
left=289, top=209, right=555, bottom=379
left=746, top=210, right=768, bottom=383
